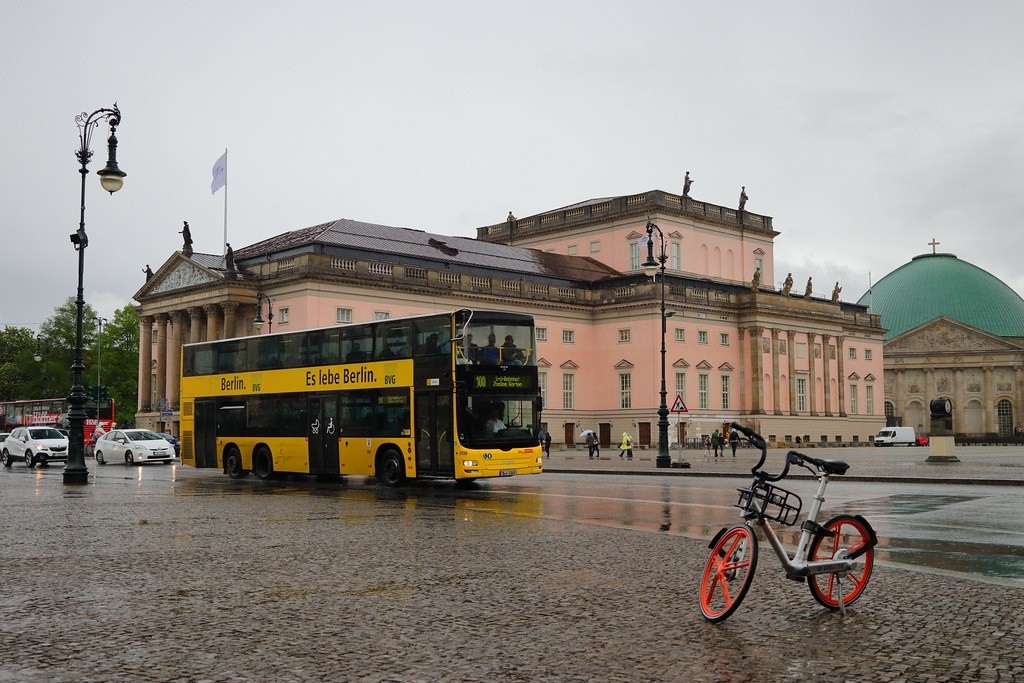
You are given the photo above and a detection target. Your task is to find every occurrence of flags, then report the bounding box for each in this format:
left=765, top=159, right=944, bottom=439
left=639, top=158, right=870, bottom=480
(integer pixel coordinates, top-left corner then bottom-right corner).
left=211, top=152, right=226, bottom=195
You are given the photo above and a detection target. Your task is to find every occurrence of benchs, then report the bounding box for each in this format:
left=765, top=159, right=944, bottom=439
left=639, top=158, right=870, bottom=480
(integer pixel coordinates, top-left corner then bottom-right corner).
left=957, top=443, right=1023, bottom=446
left=769, top=440, right=872, bottom=449
left=560, top=443, right=649, bottom=450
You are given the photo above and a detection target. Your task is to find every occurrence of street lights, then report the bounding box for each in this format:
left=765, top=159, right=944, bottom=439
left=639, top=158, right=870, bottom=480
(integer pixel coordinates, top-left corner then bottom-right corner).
left=93, top=316, right=108, bottom=426
left=62, top=101, right=128, bottom=485
left=33, top=333, right=50, bottom=398
left=640, top=215, right=671, bottom=467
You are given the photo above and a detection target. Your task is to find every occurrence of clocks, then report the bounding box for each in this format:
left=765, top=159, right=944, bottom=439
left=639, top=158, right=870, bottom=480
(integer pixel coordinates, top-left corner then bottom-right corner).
left=930, top=399, right=952, bottom=416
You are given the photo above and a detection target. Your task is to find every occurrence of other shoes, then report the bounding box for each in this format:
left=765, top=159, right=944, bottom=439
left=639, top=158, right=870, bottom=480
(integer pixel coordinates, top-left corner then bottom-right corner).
left=596, top=454, right=600, bottom=457
left=619, top=454, right=623, bottom=457
left=546, top=453, right=550, bottom=457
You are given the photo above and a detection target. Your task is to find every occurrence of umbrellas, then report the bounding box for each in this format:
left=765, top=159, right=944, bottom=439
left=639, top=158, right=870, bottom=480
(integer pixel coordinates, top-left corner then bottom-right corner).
left=580, top=430, right=593, bottom=437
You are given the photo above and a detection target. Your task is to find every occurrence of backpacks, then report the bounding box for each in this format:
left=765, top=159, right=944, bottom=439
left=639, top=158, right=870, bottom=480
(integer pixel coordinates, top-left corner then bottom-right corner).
left=626, top=440, right=631, bottom=446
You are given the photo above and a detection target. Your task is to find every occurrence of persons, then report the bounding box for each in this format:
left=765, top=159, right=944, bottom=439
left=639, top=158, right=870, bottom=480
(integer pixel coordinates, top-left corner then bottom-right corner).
left=751, top=267, right=760, bottom=291
left=488, top=409, right=507, bottom=432
left=420, top=333, right=439, bottom=353
left=705, top=428, right=742, bottom=457
left=507, top=211, right=516, bottom=221
left=456, top=334, right=524, bottom=365
left=805, top=277, right=812, bottom=298
left=539, top=428, right=551, bottom=457
left=683, top=171, right=694, bottom=195
left=225, top=243, right=234, bottom=269
left=833, top=281, right=842, bottom=300
left=586, top=432, right=599, bottom=457
left=178, top=221, right=193, bottom=246
left=142, top=265, right=153, bottom=282
left=783, top=273, right=793, bottom=295
left=738, top=186, right=748, bottom=209
left=618, top=432, right=633, bottom=458
left=92, top=421, right=118, bottom=439
left=346, top=343, right=365, bottom=361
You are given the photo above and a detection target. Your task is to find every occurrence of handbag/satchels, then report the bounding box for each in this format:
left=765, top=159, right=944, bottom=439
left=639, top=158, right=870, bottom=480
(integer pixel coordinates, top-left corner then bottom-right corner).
left=593, top=439, right=599, bottom=445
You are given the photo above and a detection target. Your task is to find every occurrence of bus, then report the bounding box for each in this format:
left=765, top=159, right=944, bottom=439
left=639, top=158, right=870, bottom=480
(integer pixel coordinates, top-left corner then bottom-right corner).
left=179, top=306, right=544, bottom=488
left=0, top=397, right=116, bottom=447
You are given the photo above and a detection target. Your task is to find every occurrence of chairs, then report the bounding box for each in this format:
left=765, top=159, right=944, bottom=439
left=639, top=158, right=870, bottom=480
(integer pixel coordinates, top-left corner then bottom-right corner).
left=363, top=412, right=389, bottom=432
left=213, top=355, right=328, bottom=373
left=344, top=349, right=394, bottom=363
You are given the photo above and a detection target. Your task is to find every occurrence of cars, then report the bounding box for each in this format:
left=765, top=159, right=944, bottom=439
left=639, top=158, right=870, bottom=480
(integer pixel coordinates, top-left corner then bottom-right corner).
left=93, top=428, right=180, bottom=466
left=0, top=426, right=70, bottom=468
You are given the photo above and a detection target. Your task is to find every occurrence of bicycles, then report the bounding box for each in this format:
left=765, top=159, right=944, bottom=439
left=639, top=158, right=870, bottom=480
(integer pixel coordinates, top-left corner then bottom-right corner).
left=698, top=421, right=878, bottom=622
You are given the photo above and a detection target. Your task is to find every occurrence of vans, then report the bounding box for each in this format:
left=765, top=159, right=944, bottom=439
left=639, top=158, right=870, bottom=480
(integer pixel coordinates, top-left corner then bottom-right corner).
left=874, top=427, right=916, bottom=447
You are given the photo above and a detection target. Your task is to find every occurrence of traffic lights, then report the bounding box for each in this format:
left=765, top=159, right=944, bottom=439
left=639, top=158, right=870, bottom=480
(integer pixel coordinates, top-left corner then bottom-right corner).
left=87, top=384, right=106, bottom=402
